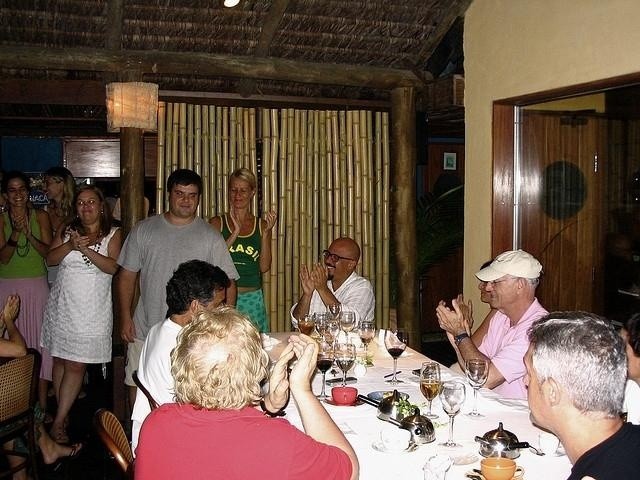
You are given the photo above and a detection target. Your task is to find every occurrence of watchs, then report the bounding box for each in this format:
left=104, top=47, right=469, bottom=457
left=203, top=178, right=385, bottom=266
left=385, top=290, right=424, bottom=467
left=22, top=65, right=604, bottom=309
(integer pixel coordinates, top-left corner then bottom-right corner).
left=454, top=332, right=470, bottom=346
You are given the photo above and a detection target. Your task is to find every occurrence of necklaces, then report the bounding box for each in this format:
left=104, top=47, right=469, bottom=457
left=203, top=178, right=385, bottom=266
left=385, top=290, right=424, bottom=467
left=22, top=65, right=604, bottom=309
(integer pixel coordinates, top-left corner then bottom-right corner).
left=8, top=206, right=30, bottom=257
left=81, top=228, right=104, bottom=267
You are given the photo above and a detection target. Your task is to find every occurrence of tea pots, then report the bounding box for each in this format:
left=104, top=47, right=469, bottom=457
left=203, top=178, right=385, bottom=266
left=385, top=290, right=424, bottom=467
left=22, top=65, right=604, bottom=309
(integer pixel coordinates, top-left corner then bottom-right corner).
left=475, top=422, right=531, bottom=459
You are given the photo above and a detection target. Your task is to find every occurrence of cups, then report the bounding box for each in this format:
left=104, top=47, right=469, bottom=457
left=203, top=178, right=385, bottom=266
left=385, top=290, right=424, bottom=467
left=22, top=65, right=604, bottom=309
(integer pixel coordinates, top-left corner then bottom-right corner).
left=314, top=339, right=333, bottom=401
left=479, top=456, right=524, bottom=480
left=331, top=385, right=357, bottom=407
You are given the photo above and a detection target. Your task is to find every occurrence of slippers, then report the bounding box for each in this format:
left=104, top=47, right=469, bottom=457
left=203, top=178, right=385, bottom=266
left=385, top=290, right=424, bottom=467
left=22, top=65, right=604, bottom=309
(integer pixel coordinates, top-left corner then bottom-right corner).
left=41, top=406, right=70, bottom=446
left=48, top=443, right=82, bottom=475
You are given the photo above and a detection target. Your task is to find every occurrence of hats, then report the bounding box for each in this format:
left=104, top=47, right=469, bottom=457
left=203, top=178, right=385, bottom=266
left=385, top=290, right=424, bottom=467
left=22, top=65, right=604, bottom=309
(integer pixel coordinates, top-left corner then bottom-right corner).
left=475, top=249, right=543, bottom=283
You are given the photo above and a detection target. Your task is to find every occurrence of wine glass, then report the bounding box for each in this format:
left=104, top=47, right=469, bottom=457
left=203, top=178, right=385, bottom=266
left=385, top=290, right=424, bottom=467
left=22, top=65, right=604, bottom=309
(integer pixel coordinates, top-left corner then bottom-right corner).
left=290, top=303, right=356, bottom=342
left=464, top=358, right=490, bottom=419
left=383, top=327, right=408, bottom=384
left=419, top=362, right=442, bottom=420
left=332, top=342, right=357, bottom=382
left=438, top=380, right=466, bottom=449
left=356, top=319, right=376, bottom=367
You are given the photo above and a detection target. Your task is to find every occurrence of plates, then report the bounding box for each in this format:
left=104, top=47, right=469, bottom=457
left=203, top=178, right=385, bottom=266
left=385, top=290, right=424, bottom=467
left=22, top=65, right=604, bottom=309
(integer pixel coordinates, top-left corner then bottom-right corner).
left=367, top=391, right=409, bottom=401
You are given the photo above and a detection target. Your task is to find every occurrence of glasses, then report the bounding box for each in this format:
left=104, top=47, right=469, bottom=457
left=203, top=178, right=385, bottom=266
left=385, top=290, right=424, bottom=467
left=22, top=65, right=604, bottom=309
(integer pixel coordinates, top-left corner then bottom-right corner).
left=77, top=200, right=104, bottom=207
left=491, top=277, right=520, bottom=288
left=322, top=251, right=357, bottom=264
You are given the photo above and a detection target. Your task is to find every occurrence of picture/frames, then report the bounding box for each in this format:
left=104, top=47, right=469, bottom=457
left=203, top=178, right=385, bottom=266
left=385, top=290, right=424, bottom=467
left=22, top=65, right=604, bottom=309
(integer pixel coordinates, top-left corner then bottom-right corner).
left=442, top=151, right=458, bottom=172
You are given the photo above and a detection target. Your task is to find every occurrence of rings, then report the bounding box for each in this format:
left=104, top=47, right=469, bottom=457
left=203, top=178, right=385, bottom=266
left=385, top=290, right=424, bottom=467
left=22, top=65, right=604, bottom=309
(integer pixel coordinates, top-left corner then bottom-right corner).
left=77, top=244, right=81, bottom=248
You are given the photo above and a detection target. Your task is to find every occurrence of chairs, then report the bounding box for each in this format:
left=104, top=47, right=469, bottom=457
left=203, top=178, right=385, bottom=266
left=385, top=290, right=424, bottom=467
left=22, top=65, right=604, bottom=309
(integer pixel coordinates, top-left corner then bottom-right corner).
left=91, top=408, right=134, bottom=478
left=0, top=347, right=41, bottom=479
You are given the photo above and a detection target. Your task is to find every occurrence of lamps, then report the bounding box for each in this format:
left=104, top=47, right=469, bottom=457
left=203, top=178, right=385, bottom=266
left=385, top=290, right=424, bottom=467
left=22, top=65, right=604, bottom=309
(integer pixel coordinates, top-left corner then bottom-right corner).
left=105, top=82, right=160, bottom=133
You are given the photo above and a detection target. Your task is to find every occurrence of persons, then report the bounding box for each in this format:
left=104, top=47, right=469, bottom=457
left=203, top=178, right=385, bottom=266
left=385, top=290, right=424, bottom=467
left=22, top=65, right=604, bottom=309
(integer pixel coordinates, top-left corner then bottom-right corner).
left=116, top=169, right=241, bottom=446
left=616, top=311, right=640, bottom=426
left=129, top=259, right=291, bottom=462
left=44, top=164, right=87, bottom=402
left=131, top=303, right=362, bottom=480
left=523, top=309, right=640, bottom=480
left=48, top=182, right=122, bottom=443
left=0, top=170, right=54, bottom=426
left=208, top=167, right=279, bottom=334
left=288, top=235, right=375, bottom=334
left=442, top=260, right=500, bottom=375
left=436, top=249, right=552, bottom=408
left=1, top=292, right=87, bottom=479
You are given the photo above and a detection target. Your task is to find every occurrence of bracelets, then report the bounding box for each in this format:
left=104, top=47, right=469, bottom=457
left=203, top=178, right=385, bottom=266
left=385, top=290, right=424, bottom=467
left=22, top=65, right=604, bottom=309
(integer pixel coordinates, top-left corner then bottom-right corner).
left=259, top=386, right=292, bottom=418
left=7, top=237, right=19, bottom=247
